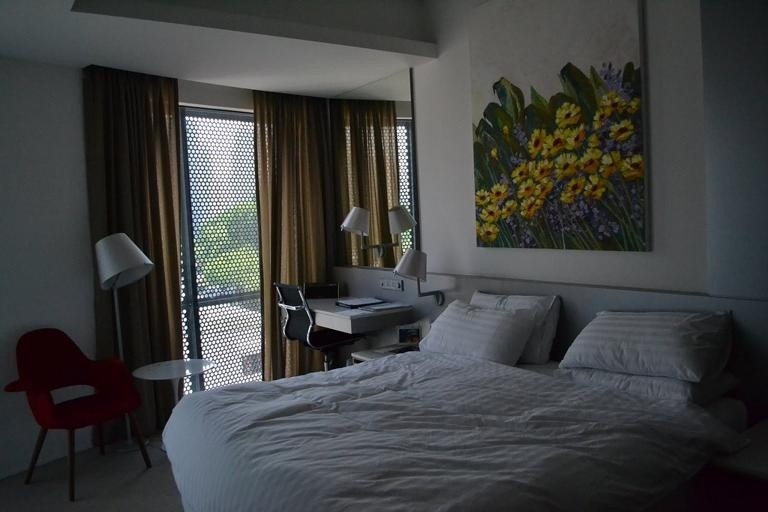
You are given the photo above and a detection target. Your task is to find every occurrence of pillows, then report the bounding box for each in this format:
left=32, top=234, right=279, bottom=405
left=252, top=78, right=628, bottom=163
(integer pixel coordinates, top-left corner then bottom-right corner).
left=417, top=299, right=537, bottom=368
left=467, top=289, right=559, bottom=365
left=558, top=308, right=735, bottom=383
left=569, top=361, right=736, bottom=413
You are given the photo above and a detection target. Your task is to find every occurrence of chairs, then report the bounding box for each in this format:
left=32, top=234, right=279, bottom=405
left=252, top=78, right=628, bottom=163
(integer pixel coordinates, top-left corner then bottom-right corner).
left=2, top=328, right=152, bottom=503
left=273, top=281, right=368, bottom=372
left=303, top=282, right=340, bottom=300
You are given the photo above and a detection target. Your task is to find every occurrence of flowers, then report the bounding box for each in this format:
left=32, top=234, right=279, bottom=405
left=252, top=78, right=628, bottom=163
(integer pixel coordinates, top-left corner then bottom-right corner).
left=471, top=0, right=647, bottom=252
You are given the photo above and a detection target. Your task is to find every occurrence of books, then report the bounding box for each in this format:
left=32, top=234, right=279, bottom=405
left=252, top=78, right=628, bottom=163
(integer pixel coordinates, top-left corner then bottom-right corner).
left=337, top=296, right=411, bottom=314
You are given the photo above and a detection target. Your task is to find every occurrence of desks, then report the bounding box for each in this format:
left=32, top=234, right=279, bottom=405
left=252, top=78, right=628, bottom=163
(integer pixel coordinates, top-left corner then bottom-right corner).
left=280, top=296, right=413, bottom=334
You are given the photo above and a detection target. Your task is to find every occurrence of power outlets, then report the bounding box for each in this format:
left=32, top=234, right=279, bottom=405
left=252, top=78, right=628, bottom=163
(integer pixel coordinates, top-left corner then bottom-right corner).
left=377, top=278, right=403, bottom=292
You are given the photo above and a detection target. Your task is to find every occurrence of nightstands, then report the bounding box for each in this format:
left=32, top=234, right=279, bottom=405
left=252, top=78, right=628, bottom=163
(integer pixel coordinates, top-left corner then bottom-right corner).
left=350, top=345, right=419, bottom=366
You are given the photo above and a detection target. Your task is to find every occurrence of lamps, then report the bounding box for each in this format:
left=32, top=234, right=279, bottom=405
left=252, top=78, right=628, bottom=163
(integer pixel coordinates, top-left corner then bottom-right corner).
left=391, top=248, right=443, bottom=306
left=339, top=207, right=380, bottom=258
left=95, top=231, right=155, bottom=454
left=382, top=205, right=417, bottom=258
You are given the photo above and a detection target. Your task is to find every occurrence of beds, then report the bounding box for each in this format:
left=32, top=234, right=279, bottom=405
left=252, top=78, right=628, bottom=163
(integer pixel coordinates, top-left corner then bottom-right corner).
left=161, top=350, right=750, bottom=511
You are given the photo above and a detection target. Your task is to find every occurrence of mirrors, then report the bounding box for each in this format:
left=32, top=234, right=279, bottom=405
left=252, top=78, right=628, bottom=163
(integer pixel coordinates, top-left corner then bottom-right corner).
left=329, top=68, right=417, bottom=270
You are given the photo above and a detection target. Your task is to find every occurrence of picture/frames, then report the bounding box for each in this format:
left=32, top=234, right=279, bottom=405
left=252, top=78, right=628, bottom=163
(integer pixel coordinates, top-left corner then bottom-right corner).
left=396, top=325, right=421, bottom=346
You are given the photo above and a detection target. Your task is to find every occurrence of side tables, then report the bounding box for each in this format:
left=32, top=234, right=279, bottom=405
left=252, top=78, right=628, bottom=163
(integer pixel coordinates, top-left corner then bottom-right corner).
left=131, top=359, right=215, bottom=453
